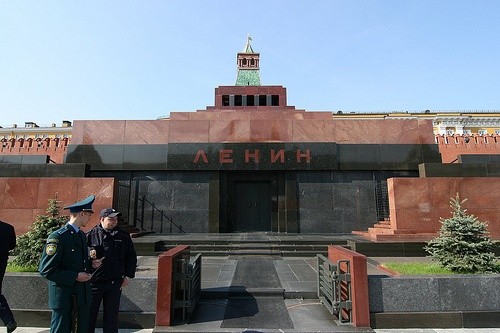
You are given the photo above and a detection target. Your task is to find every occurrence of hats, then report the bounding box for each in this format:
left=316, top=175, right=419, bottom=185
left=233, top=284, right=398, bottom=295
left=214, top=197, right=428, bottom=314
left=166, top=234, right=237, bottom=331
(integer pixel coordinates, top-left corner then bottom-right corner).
left=63, top=195, right=95, bottom=213
left=100, top=207, right=122, bottom=218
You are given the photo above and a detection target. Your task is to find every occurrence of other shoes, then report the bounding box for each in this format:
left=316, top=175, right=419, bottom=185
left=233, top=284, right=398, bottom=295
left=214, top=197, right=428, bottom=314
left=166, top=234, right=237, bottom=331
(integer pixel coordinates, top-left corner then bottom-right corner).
left=7, top=320, right=17, bottom=333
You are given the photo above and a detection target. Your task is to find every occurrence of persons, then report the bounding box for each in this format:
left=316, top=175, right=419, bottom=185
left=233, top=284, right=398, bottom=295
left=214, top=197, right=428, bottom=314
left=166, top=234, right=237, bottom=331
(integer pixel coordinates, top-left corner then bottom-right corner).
left=86, top=208, right=137, bottom=333
left=38, top=195, right=102, bottom=333
left=0, top=221, right=17, bottom=333
